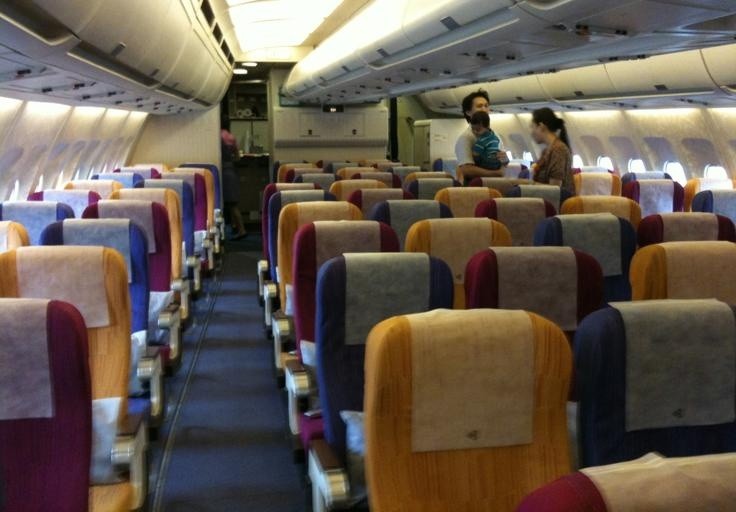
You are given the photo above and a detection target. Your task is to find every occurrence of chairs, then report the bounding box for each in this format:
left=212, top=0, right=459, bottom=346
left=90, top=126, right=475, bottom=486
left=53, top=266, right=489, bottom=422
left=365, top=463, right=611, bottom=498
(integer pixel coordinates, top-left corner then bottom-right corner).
left=258, top=158, right=735, bottom=511
left=1, top=162, right=225, bottom=510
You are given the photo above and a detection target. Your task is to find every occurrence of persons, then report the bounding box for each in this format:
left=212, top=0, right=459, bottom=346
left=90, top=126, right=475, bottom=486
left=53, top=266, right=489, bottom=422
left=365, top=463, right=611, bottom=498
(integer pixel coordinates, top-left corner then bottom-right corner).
left=463, top=111, right=502, bottom=187
left=531, top=107, right=576, bottom=201
left=220, top=126, right=247, bottom=239
left=454, top=87, right=509, bottom=187
